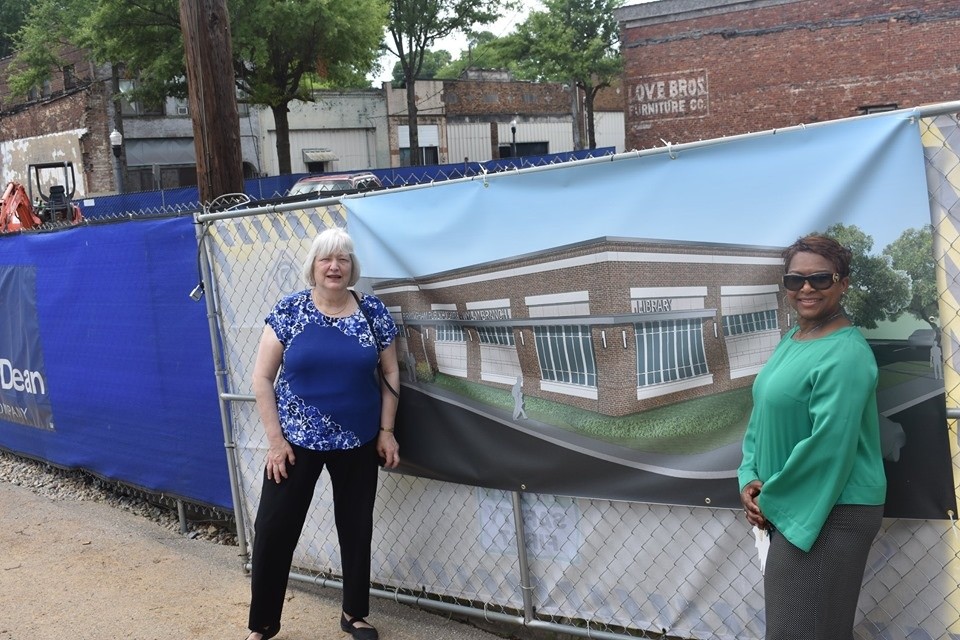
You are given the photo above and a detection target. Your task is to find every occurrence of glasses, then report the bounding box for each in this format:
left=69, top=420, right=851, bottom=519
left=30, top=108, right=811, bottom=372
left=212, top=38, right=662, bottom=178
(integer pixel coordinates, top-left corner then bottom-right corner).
left=782, top=272, right=838, bottom=290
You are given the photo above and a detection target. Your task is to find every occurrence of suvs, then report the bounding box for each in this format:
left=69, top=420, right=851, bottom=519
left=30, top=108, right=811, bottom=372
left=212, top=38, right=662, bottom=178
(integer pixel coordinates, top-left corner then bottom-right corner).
left=287, top=171, right=383, bottom=197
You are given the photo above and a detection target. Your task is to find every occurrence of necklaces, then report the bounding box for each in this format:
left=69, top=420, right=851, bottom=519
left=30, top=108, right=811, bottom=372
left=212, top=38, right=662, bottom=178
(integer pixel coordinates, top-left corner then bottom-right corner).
left=796, top=312, right=844, bottom=341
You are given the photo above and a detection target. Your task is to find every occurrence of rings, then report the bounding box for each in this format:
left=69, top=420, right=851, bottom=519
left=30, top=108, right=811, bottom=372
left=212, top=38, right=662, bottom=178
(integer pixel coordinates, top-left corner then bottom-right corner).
left=743, top=510, right=748, bottom=517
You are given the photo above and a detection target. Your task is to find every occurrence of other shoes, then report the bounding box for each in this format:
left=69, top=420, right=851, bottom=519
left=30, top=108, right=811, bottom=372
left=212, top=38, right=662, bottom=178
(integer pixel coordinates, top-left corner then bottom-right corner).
left=243, top=621, right=281, bottom=640
left=340, top=609, right=379, bottom=640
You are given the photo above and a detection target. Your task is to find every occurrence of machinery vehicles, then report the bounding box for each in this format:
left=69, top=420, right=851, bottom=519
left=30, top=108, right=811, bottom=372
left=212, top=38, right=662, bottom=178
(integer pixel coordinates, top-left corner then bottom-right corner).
left=0, top=160, right=82, bottom=233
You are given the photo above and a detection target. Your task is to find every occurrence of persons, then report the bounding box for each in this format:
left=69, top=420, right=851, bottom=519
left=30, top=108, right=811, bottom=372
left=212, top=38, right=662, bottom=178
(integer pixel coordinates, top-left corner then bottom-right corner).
left=738, top=235, right=888, bottom=640
left=245, top=228, right=402, bottom=640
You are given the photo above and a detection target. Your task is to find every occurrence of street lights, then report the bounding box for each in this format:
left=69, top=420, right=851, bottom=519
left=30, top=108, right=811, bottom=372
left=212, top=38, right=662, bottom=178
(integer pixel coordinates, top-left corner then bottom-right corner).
left=510, top=119, right=516, bottom=157
left=109, top=128, right=124, bottom=194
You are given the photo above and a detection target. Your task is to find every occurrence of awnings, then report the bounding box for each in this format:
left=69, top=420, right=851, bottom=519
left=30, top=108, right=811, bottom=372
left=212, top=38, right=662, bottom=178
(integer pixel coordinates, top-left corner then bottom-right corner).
left=302, top=148, right=339, bottom=163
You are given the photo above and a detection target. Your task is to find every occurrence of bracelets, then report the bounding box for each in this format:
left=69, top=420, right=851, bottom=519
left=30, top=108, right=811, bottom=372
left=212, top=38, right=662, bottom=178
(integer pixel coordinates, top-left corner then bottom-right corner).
left=380, top=426, right=394, bottom=432
